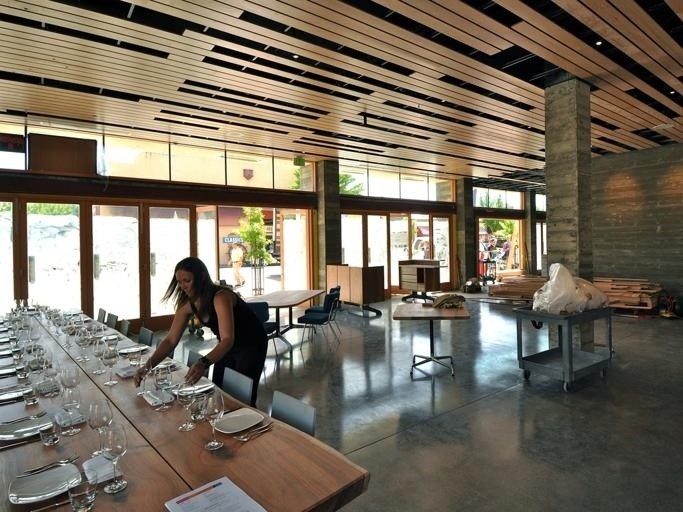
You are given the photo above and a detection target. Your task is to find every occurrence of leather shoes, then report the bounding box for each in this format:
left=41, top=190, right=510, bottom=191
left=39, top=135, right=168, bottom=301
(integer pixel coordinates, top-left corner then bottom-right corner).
left=242, top=281, right=245, bottom=285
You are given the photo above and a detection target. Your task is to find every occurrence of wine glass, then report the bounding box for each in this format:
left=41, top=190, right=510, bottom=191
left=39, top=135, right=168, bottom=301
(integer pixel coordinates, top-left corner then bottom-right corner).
left=200, top=392, right=226, bottom=452
left=87, top=400, right=113, bottom=459
left=43, top=357, right=61, bottom=382
left=136, top=355, right=152, bottom=395
left=103, top=347, right=120, bottom=387
left=61, top=365, right=80, bottom=400
left=153, top=365, right=173, bottom=414
left=61, top=387, right=81, bottom=437
left=175, top=380, right=198, bottom=431
left=101, top=424, right=129, bottom=494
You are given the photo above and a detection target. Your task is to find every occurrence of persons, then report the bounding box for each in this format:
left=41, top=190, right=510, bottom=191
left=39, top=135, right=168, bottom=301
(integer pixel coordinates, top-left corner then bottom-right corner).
left=133, top=256, right=270, bottom=409
left=487, top=238, right=497, bottom=277
left=501, top=235, right=511, bottom=260
left=227, top=241, right=247, bottom=289
left=422, top=239, right=431, bottom=260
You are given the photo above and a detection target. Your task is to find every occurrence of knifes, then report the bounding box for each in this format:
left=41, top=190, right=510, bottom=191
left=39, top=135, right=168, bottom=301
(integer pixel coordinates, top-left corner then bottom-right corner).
left=15, top=455, right=81, bottom=478
left=1, top=409, right=47, bottom=426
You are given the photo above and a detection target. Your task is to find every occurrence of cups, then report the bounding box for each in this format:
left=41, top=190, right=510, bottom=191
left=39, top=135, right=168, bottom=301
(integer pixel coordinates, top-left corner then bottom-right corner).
left=65, top=470, right=97, bottom=512
left=37, top=423, right=61, bottom=448
left=128, top=345, right=142, bottom=368
left=188, top=393, right=212, bottom=425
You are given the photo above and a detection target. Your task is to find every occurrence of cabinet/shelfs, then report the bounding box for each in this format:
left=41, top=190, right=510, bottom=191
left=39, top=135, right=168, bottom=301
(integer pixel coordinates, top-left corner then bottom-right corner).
left=327, top=264, right=385, bottom=315
left=399, top=260, right=440, bottom=303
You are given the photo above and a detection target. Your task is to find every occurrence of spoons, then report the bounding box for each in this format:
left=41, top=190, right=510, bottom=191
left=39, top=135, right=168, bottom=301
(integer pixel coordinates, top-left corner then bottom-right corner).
left=232, top=421, right=273, bottom=442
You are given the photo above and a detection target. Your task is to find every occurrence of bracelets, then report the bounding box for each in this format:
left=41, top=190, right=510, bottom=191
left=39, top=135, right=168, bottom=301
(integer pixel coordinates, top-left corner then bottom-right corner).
left=200, top=356, right=212, bottom=365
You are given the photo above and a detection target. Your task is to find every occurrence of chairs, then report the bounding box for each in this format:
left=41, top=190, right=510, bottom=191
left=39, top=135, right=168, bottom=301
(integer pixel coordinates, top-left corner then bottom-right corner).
left=98, top=308, right=316, bottom=437
left=305, top=286, right=342, bottom=339
left=246, top=302, right=293, bottom=380
left=298, top=290, right=340, bottom=352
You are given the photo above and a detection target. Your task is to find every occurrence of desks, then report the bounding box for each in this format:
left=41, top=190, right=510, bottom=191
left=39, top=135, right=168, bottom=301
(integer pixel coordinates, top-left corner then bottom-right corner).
left=247, top=290, right=326, bottom=354
left=393, top=298, right=470, bottom=376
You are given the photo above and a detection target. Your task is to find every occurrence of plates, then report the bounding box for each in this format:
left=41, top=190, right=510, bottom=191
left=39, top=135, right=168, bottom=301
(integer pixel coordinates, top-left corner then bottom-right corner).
left=0, top=417, right=53, bottom=441
left=118, top=345, right=150, bottom=353
left=2, top=383, right=32, bottom=401
left=211, top=407, right=265, bottom=434
left=168, top=375, right=215, bottom=396
left=7, top=463, right=82, bottom=505
